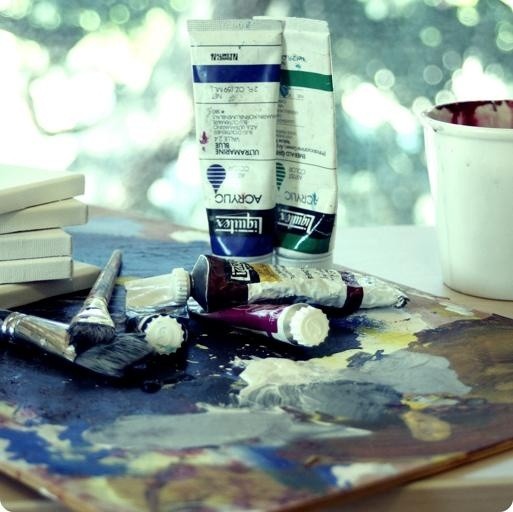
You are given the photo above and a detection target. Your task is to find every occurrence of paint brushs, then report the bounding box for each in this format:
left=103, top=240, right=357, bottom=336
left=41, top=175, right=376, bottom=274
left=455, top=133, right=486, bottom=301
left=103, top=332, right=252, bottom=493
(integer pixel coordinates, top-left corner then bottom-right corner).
left=0, top=248, right=156, bottom=378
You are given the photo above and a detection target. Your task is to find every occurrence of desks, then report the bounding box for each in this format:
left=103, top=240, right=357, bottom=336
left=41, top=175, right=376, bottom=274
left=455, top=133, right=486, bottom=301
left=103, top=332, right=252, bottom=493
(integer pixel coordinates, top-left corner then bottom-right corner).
left=0, top=205, right=513, bottom=512
